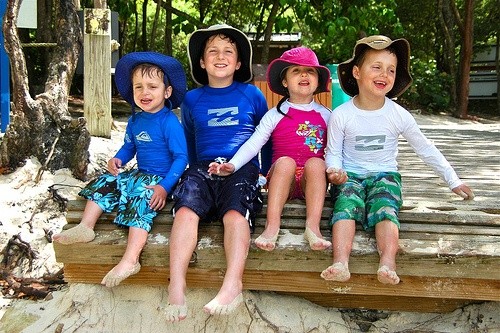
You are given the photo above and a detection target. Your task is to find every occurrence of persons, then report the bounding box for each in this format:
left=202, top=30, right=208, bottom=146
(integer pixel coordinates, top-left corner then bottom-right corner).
left=166, top=24, right=274, bottom=322
left=207, top=47, right=332, bottom=251
left=321, top=35, right=475, bottom=284
left=52, top=51, right=189, bottom=287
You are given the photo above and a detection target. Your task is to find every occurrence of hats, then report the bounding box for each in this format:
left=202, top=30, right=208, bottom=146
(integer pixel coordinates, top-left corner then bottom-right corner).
left=114, top=52, right=188, bottom=109
left=337, top=35, right=413, bottom=99
left=187, top=24, right=253, bottom=87
left=266, top=46, right=331, bottom=96
